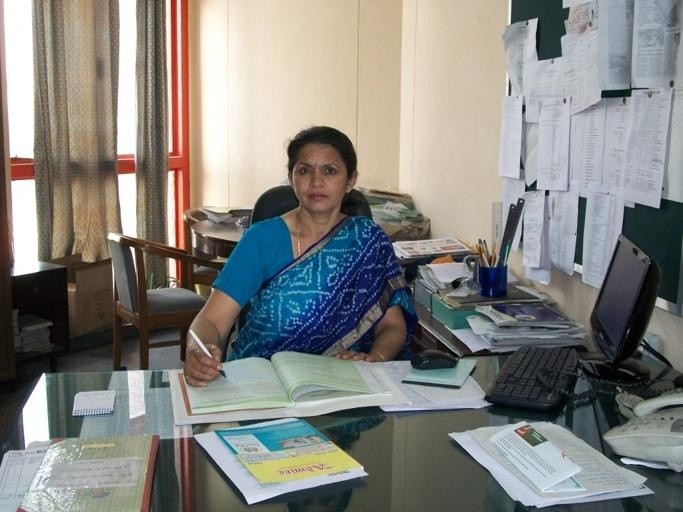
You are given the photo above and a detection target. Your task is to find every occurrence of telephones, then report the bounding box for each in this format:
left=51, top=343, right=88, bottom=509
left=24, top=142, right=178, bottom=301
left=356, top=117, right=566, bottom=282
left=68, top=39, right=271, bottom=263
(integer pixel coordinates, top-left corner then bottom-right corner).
left=603, top=394, right=683, bottom=472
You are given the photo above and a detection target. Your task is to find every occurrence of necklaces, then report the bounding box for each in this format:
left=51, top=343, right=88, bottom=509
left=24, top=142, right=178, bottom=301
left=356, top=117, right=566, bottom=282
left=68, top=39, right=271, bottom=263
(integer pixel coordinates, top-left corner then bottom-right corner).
left=295, top=210, right=303, bottom=261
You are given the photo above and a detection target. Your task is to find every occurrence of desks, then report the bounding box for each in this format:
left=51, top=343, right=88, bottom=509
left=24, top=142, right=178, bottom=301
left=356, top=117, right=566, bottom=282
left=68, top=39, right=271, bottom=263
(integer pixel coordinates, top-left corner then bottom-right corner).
left=410, top=292, right=492, bottom=357
left=192, top=218, right=244, bottom=245
left=0, top=352, right=683, bottom=512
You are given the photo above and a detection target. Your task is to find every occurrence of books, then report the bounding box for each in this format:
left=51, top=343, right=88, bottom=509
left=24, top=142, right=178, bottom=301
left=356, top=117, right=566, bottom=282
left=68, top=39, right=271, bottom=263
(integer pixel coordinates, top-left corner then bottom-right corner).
left=13, top=304, right=54, bottom=353
left=15, top=431, right=159, bottom=511
left=70, top=388, right=116, bottom=417
left=177, top=348, right=392, bottom=415
left=193, top=417, right=367, bottom=504
left=395, top=234, right=587, bottom=389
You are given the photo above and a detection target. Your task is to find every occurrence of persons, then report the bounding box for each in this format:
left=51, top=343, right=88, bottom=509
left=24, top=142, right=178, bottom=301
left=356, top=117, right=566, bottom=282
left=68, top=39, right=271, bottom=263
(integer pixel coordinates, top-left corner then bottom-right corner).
left=183, top=126, right=420, bottom=388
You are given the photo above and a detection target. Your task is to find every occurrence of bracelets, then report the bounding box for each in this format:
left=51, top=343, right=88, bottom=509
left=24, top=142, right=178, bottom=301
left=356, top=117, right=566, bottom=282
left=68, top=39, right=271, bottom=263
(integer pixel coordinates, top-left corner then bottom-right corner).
left=378, top=353, right=385, bottom=362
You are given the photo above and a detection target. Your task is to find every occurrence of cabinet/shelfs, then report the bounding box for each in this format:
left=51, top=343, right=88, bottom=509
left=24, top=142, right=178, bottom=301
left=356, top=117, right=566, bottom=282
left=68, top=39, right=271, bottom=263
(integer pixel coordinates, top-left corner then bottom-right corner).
left=9, top=259, right=69, bottom=373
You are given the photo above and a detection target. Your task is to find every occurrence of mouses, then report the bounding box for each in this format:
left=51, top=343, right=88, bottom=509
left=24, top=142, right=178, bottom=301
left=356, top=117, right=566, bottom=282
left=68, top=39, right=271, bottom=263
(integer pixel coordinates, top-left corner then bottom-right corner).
left=410, top=349, right=458, bottom=370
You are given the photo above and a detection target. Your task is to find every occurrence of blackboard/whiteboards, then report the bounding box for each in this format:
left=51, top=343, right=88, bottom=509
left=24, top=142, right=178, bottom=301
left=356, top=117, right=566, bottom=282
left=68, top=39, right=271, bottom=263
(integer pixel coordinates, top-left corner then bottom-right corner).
left=506, top=0, right=683, bottom=315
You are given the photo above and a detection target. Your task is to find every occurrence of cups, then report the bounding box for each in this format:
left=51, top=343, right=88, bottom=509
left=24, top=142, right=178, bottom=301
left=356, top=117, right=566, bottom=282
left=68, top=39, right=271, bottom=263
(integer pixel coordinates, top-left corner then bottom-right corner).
left=463, top=255, right=493, bottom=283
left=478, top=264, right=508, bottom=299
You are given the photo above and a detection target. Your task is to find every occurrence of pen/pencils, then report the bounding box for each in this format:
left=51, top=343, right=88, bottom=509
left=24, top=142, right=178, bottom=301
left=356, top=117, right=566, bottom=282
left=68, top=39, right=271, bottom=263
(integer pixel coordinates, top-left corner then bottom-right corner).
left=476, top=239, right=510, bottom=268
left=189, top=330, right=226, bottom=378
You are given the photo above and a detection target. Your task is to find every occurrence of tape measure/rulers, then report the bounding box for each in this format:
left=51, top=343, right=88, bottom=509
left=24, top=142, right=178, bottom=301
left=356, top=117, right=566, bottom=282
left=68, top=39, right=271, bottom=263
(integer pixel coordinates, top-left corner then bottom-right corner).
left=499, top=198, right=525, bottom=264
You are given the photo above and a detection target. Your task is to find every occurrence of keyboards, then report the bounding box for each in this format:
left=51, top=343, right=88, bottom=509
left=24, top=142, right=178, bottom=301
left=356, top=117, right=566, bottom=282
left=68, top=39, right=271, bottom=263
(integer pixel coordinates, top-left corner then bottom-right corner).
left=482, top=345, right=580, bottom=413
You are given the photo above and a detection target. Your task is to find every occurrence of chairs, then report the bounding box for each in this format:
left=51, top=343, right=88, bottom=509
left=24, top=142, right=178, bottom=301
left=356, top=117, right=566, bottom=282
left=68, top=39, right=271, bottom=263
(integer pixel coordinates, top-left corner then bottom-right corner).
left=108, top=232, right=225, bottom=370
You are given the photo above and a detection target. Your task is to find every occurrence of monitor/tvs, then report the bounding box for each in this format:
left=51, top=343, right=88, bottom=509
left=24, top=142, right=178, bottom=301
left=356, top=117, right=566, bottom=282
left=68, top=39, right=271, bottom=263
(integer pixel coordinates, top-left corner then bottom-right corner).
left=579, top=233, right=662, bottom=385
